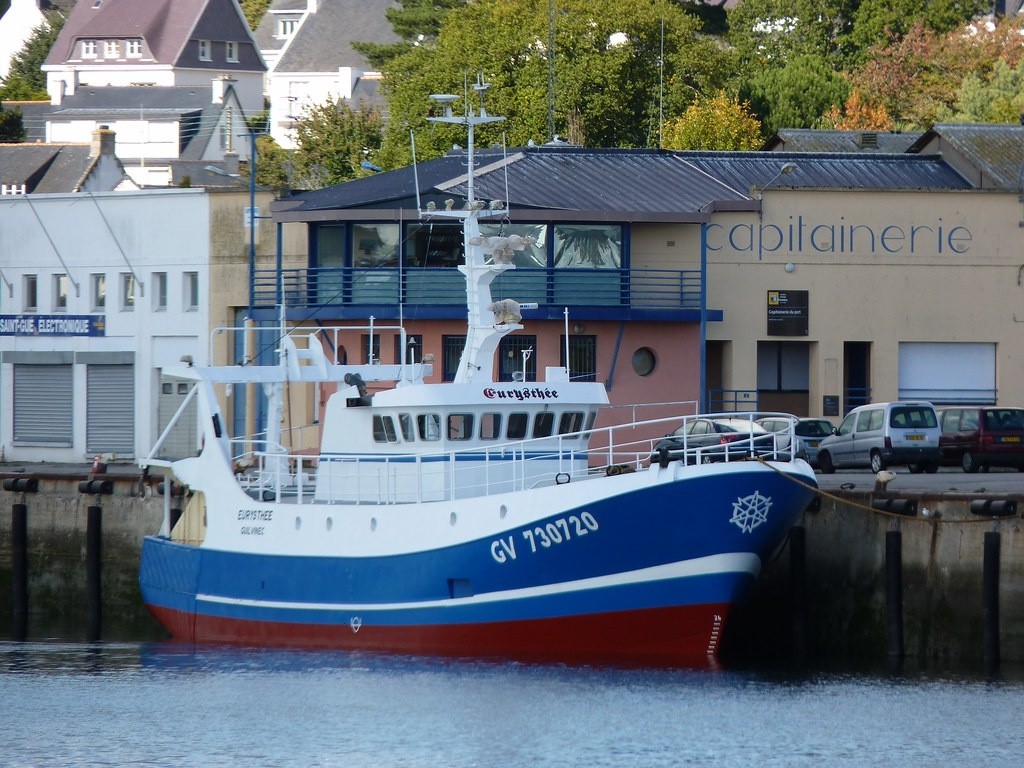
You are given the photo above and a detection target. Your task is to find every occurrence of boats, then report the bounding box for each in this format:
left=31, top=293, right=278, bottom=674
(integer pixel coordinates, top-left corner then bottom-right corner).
left=136, top=67, right=818, bottom=675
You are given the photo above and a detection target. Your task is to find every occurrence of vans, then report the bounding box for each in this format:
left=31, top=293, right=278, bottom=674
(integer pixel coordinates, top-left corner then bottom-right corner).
left=818, top=400, right=943, bottom=474
left=907, top=406, right=1023, bottom=473
left=755, top=418, right=836, bottom=472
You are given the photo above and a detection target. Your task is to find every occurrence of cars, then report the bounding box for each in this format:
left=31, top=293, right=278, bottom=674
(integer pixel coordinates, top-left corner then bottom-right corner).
left=650, top=418, right=779, bottom=466
